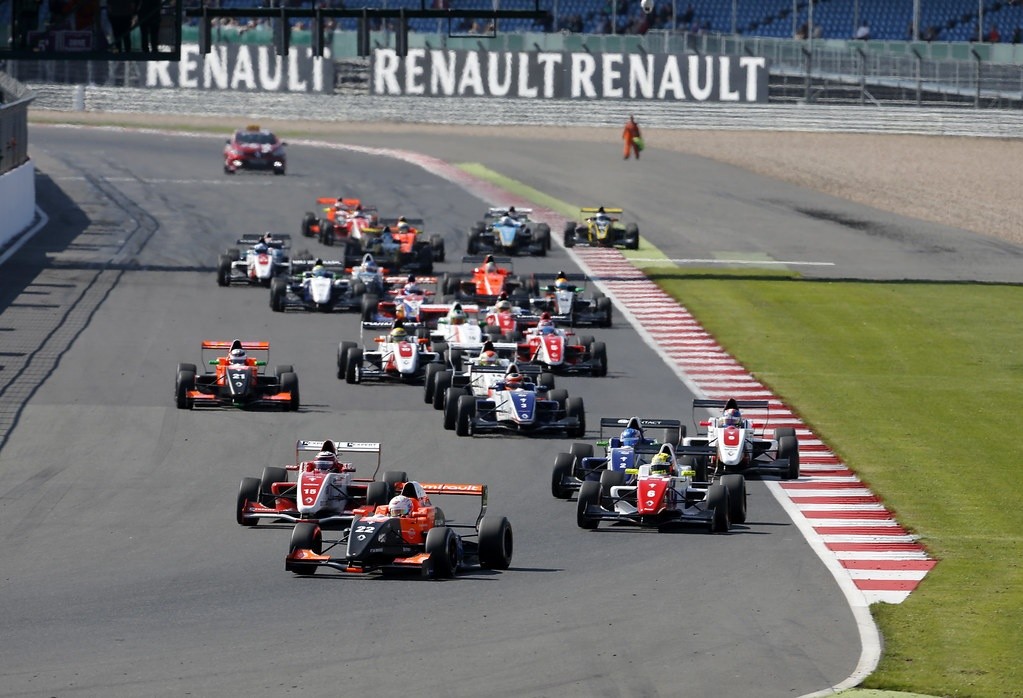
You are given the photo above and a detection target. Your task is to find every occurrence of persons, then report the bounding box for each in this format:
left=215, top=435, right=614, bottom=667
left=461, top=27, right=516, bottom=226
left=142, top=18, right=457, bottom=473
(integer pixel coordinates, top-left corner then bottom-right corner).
left=253, top=243, right=268, bottom=254
left=366, top=214, right=378, bottom=228
left=397, top=221, right=409, bottom=234
left=505, top=373, right=525, bottom=390
left=496, top=300, right=513, bottom=313
left=364, top=260, right=377, bottom=273
left=479, top=350, right=501, bottom=367
left=620, top=409, right=742, bottom=477
left=538, top=319, right=556, bottom=335
left=484, top=261, right=499, bottom=273
left=621, top=114, right=640, bottom=160
left=498, top=216, right=512, bottom=226
left=314, top=450, right=342, bottom=473
left=388, top=495, right=413, bottom=517
left=404, top=282, right=420, bottom=295
left=311, top=265, right=327, bottom=278
left=228, top=349, right=247, bottom=365
left=448, top=309, right=466, bottom=324
left=183, top=0, right=1023, bottom=44
left=390, top=327, right=408, bottom=343
left=596, top=212, right=607, bottom=226
left=554, top=278, right=569, bottom=292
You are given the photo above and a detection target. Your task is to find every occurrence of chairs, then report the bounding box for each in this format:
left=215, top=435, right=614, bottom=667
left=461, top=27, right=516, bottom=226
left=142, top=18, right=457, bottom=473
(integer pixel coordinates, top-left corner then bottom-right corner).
left=130, top=0, right=1023, bottom=65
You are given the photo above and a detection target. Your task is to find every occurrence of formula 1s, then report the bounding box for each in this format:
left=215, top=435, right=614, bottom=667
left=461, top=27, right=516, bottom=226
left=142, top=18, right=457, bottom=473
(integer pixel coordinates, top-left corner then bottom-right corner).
left=551, top=398, right=800, bottom=534
left=175, top=339, right=300, bottom=412
left=563, top=207, right=639, bottom=249
left=466, top=207, right=552, bottom=256
left=237, top=440, right=514, bottom=580
left=217, top=197, right=612, bottom=438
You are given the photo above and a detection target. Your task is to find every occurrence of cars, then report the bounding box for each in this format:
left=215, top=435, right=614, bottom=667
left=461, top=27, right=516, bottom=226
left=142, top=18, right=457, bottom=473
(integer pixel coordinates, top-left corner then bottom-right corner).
left=223, top=125, right=288, bottom=175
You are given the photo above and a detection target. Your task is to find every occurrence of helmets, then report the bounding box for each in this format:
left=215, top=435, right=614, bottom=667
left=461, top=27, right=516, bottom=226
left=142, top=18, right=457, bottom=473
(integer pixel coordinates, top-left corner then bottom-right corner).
left=452, top=310, right=466, bottom=323
left=397, top=221, right=409, bottom=234
left=595, top=212, right=610, bottom=225
left=553, top=279, right=568, bottom=291
left=388, top=496, right=413, bottom=516
left=650, top=452, right=673, bottom=475
left=253, top=243, right=268, bottom=254
left=228, top=349, right=247, bottom=367
left=353, top=209, right=364, bottom=218
left=497, top=300, right=512, bottom=315
left=390, top=326, right=408, bottom=342
left=479, top=351, right=498, bottom=366
left=314, top=450, right=337, bottom=472
left=619, top=429, right=641, bottom=448
left=500, top=217, right=513, bottom=225
left=721, top=409, right=742, bottom=427
left=485, top=262, right=498, bottom=274
left=504, top=371, right=524, bottom=390
left=405, top=282, right=420, bottom=295
left=364, top=262, right=378, bottom=274
left=537, top=320, right=555, bottom=336
left=312, top=266, right=328, bottom=278
